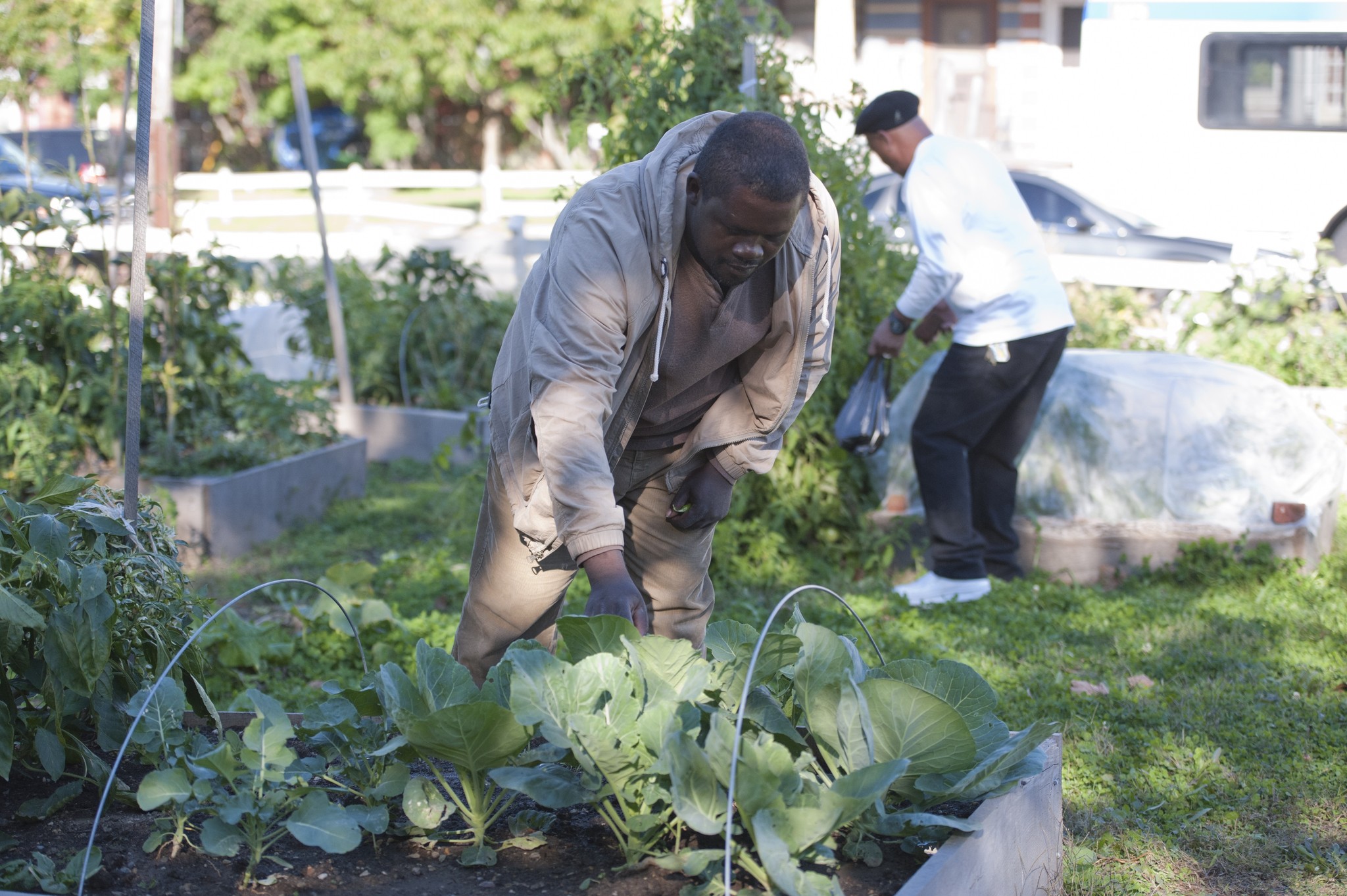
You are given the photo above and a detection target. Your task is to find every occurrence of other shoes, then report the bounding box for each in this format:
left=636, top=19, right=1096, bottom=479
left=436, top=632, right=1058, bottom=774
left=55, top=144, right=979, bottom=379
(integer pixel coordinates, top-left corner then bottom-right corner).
left=890, top=569, right=992, bottom=606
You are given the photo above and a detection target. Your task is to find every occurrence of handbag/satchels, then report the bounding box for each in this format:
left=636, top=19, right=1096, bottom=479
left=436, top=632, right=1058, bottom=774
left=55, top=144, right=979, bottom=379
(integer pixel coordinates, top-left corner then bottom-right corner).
left=833, top=353, right=892, bottom=459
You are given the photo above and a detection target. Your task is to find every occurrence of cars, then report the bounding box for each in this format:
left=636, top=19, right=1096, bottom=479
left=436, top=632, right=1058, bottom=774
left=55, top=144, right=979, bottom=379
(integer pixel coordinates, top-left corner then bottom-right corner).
left=851, top=168, right=1262, bottom=283
left=0, top=133, right=137, bottom=227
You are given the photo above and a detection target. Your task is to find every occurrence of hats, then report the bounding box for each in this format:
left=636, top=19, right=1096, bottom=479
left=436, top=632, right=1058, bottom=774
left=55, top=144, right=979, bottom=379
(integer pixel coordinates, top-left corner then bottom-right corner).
left=854, top=90, right=919, bottom=136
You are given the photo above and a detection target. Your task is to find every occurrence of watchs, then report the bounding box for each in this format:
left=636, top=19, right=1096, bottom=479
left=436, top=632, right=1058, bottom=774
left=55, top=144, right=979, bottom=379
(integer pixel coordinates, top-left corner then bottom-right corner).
left=889, top=312, right=910, bottom=335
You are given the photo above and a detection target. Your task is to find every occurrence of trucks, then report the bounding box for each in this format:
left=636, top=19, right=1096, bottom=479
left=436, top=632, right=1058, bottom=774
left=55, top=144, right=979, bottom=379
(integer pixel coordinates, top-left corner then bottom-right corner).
left=1080, top=2, right=1347, bottom=268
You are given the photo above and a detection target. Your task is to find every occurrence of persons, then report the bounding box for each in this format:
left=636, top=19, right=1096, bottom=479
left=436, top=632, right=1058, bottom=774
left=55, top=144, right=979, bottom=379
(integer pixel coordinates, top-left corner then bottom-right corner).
left=453, top=114, right=841, bottom=692
left=854, top=91, right=1073, bottom=605
